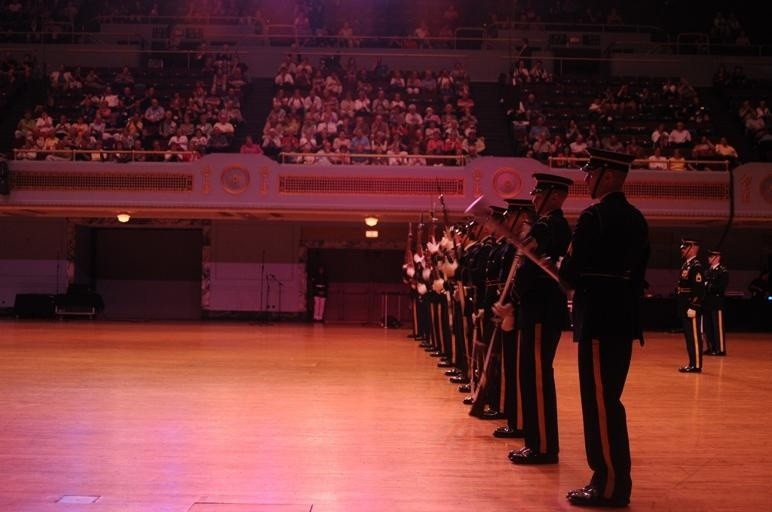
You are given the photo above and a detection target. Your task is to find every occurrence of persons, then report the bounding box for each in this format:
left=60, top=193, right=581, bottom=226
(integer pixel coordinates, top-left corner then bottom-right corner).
left=311, top=265, right=329, bottom=323
left=14, top=45, right=251, bottom=159
left=262, top=54, right=485, bottom=164
left=401, top=198, right=542, bottom=438
left=507, top=58, right=739, bottom=170
left=555, top=145, right=653, bottom=508
left=153, top=2, right=698, bottom=57
left=674, top=232, right=712, bottom=374
left=744, top=272, right=770, bottom=332
left=702, top=247, right=730, bottom=357
left=506, top=178, right=574, bottom=466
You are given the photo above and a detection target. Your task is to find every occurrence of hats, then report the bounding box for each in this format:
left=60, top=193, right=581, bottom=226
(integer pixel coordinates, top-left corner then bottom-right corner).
left=530, top=173, right=573, bottom=195
left=706, top=248, right=720, bottom=256
left=488, top=205, right=507, bottom=221
left=503, top=198, right=533, bottom=216
left=680, top=238, right=698, bottom=248
left=453, top=217, right=479, bottom=236
left=580, top=146, right=637, bottom=174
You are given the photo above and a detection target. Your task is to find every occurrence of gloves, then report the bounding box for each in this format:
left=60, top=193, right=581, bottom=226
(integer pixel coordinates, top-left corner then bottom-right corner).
left=687, top=308, right=696, bottom=317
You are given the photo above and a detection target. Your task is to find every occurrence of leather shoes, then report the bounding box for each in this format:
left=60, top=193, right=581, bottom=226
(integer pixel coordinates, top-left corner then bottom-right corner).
left=508, top=446, right=560, bottom=464
left=704, top=349, right=725, bottom=356
left=679, top=366, right=701, bottom=372
left=406, top=333, right=524, bottom=438
left=568, top=485, right=629, bottom=507
